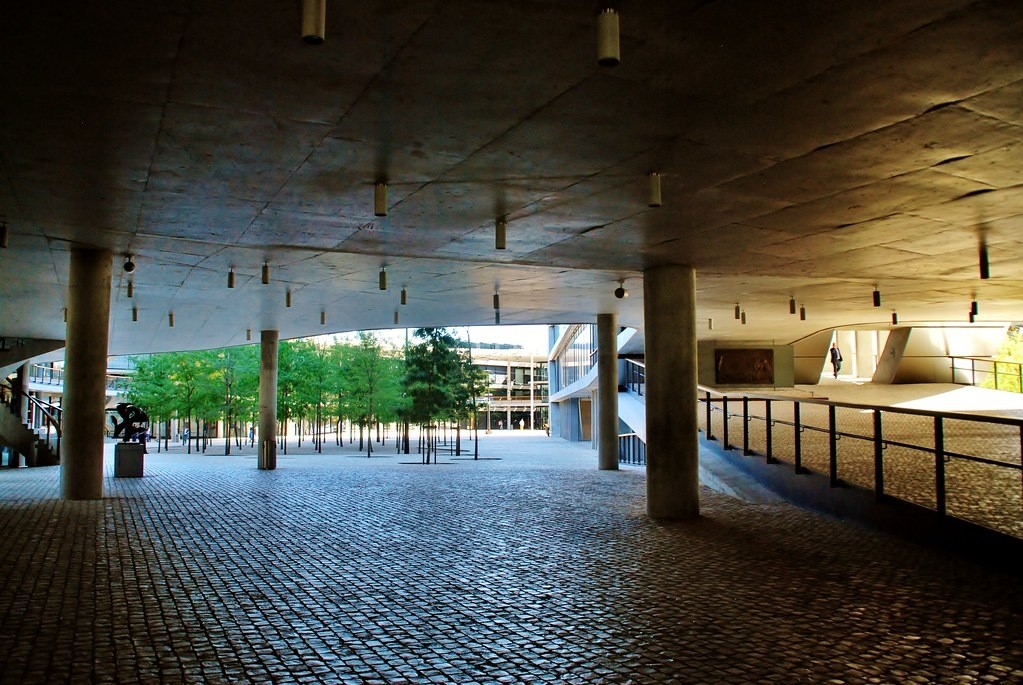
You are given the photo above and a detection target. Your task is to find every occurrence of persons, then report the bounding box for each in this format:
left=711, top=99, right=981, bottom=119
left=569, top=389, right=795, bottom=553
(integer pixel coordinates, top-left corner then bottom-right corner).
left=132, top=423, right=150, bottom=442
left=830, top=342, right=843, bottom=379
left=184, top=429, right=189, bottom=443
left=519, top=418, right=525, bottom=430
left=249, top=427, right=253, bottom=443
left=499, top=420, right=503, bottom=429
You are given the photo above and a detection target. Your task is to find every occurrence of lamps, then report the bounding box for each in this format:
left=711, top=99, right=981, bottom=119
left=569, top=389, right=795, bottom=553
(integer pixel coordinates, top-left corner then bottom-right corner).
left=706, top=243, right=992, bottom=331
left=63, top=309, right=67, bottom=321
left=492, top=291, right=500, bottom=309
left=0, top=222, right=9, bottom=248
left=261, top=261, right=270, bottom=284
left=247, top=330, right=250, bottom=340
left=495, top=218, right=507, bottom=249
left=646, top=173, right=661, bottom=208
left=126, top=281, right=134, bottom=297
left=285, top=292, right=292, bottom=307
left=227, top=266, right=234, bottom=289
left=300, top=0, right=326, bottom=44
left=595, top=8, right=621, bottom=66
left=380, top=266, right=387, bottom=290
left=401, top=286, right=410, bottom=306
left=321, top=312, right=325, bottom=324
left=395, top=311, right=400, bottom=324
left=132, top=308, right=137, bottom=323
left=373, top=183, right=388, bottom=216
left=169, top=314, right=174, bottom=327
